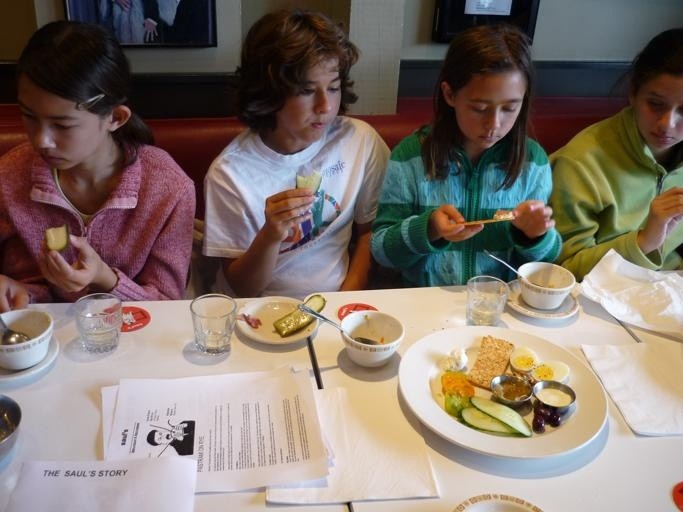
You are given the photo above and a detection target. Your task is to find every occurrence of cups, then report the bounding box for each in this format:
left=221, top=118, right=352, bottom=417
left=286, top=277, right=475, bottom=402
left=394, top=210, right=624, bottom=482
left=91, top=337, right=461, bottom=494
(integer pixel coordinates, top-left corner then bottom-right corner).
left=189, top=294, right=238, bottom=356
left=466, top=275, right=507, bottom=326
left=74, top=292, right=121, bottom=352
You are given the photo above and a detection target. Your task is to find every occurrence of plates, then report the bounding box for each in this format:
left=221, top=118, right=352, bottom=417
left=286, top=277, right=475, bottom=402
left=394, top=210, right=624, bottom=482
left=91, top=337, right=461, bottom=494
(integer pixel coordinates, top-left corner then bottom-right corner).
left=0, top=331, right=62, bottom=385
left=507, top=279, right=582, bottom=322
left=232, top=296, right=322, bottom=347
left=397, top=326, right=612, bottom=459
left=450, top=493, right=544, bottom=512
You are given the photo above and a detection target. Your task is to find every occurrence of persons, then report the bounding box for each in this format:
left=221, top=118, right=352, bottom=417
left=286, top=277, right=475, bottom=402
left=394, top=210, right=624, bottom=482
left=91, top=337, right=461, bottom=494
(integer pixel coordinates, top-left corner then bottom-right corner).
left=370, top=25, right=564, bottom=289
left=145, top=419, right=196, bottom=458
left=201, top=10, right=391, bottom=298
left=549, top=26, right=683, bottom=284
left=0, top=19, right=196, bottom=316
left=92, top=1, right=146, bottom=43
left=144, top=1, right=209, bottom=45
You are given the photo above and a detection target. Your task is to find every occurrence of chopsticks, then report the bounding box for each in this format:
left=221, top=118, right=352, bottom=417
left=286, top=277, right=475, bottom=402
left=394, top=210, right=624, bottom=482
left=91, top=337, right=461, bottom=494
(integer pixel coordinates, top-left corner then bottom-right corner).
left=307, top=337, right=323, bottom=388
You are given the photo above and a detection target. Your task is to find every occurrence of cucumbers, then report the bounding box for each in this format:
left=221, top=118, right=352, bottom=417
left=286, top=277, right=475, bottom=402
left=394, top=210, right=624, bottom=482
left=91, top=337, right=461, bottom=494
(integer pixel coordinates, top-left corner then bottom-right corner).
left=273, top=294, right=325, bottom=337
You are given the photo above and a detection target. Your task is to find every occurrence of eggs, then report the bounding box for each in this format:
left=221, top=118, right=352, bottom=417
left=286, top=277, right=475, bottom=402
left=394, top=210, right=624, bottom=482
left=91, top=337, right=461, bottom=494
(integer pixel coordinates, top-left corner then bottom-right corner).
left=510, top=350, right=537, bottom=370
left=531, top=359, right=568, bottom=382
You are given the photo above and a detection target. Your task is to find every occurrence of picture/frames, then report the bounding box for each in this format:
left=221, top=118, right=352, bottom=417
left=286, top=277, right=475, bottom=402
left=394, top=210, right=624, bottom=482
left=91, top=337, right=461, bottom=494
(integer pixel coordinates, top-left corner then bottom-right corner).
left=62, top=0, right=219, bottom=52
left=429, top=1, right=540, bottom=48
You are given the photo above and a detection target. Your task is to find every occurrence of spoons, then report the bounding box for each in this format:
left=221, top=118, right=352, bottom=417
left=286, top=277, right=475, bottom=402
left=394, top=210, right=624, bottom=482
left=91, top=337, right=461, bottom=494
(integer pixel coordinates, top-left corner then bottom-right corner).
left=0, top=319, right=31, bottom=346
left=483, top=249, right=544, bottom=288
left=297, top=302, right=379, bottom=346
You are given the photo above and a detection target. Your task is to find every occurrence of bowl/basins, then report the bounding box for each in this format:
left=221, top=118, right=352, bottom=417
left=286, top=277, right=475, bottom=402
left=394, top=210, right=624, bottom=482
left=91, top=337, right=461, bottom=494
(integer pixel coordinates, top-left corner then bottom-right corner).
left=518, top=261, right=576, bottom=310
left=338, top=310, right=404, bottom=370
left=0, top=307, right=55, bottom=372
left=0, top=394, right=22, bottom=463
